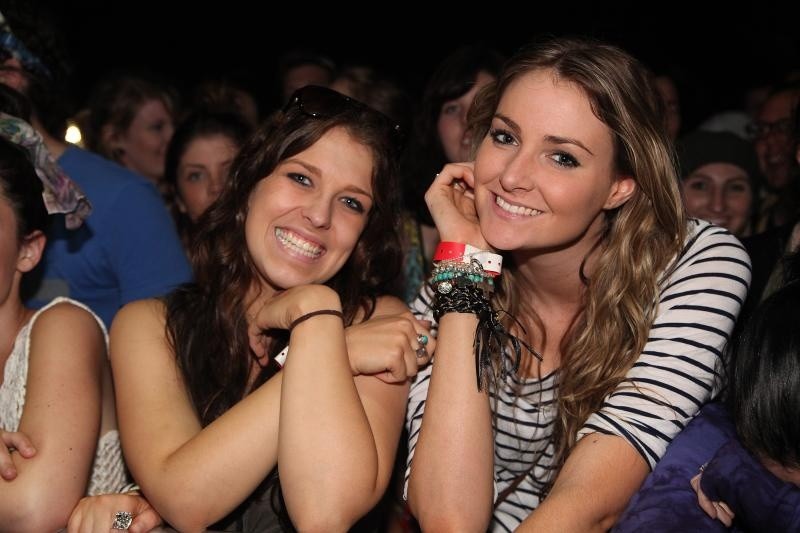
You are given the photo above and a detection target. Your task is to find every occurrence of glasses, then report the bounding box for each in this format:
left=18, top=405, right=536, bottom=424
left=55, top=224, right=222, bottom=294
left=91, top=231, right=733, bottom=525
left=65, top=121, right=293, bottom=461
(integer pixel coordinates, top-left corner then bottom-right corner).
left=744, top=117, right=793, bottom=141
left=273, top=84, right=401, bottom=167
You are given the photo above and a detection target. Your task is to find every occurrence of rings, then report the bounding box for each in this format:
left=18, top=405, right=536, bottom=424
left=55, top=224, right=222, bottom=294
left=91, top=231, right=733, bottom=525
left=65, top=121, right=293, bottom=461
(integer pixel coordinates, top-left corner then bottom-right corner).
left=416, top=347, right=427, bottom=357
left=416, top=334, right=428, bottom=346
left=112, top=510, right=133, bottom=530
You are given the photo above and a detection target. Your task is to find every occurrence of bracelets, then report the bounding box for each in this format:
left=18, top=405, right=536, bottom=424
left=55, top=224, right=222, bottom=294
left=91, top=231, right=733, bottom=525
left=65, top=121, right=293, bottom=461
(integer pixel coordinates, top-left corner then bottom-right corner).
left=431, top=274, right=494, bottom=286
left=430, top=284, right=540, bottom=381
left=288, top=310, right=345, bottom=332
left=431, top=240, right=502, bottom=275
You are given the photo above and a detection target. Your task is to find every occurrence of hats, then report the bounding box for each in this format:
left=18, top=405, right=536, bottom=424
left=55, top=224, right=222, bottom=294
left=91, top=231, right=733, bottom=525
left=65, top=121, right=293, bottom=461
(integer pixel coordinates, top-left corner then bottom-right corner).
left=677, top=131, right=760, bottom=181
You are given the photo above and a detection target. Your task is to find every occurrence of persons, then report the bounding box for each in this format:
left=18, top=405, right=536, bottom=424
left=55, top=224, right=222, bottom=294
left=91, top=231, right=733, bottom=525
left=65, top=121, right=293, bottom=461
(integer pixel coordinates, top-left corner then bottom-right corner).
left=0, top=0, right=800, bottom=533
left=110, top=83, right=436, bottom=533
left=0, top=108, right=129, bottom=533
left=69, top=33, right=753, bottom=533
